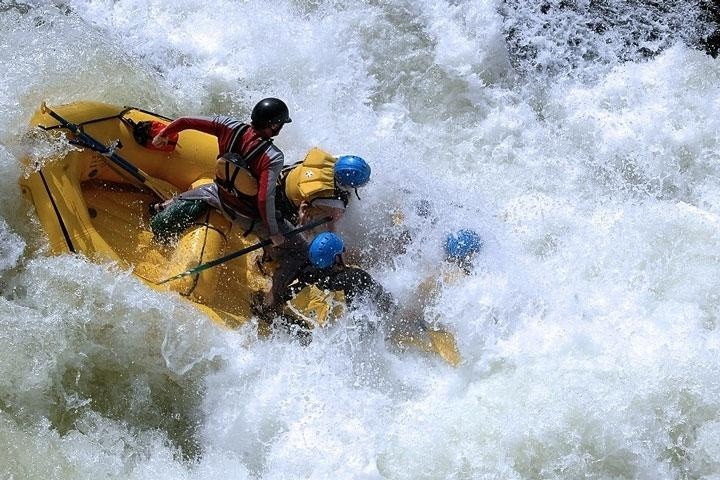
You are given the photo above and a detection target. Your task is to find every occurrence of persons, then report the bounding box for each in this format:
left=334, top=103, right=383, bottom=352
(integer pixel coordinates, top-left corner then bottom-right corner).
left=276, top=148, right=371, bottom=236
left=283, top=230, right=483, bottom=347
left=150, top=98, right=309, bottom=328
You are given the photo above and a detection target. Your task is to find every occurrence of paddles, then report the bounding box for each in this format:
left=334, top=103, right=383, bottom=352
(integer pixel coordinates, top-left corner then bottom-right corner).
left=414, top=316, right=463, bottom=368
left=39, top=102, right=183, bottom=201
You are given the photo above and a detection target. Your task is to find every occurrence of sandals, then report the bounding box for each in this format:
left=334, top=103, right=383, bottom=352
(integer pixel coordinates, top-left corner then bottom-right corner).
left=149, top=201, right=165, bottom=216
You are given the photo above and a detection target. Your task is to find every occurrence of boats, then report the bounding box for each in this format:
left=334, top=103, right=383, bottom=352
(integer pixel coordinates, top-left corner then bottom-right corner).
left=16, top=99, right=460, bottom=371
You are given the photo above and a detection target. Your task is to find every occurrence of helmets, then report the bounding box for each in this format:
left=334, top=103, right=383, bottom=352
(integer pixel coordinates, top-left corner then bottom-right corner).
left=335, top=156, right=371, bottom=188
left=446, top=230, right=482, bottom=257
left=307, top=230, right=344, bottom=268
left=252, top=98, right=292, bottom=123
